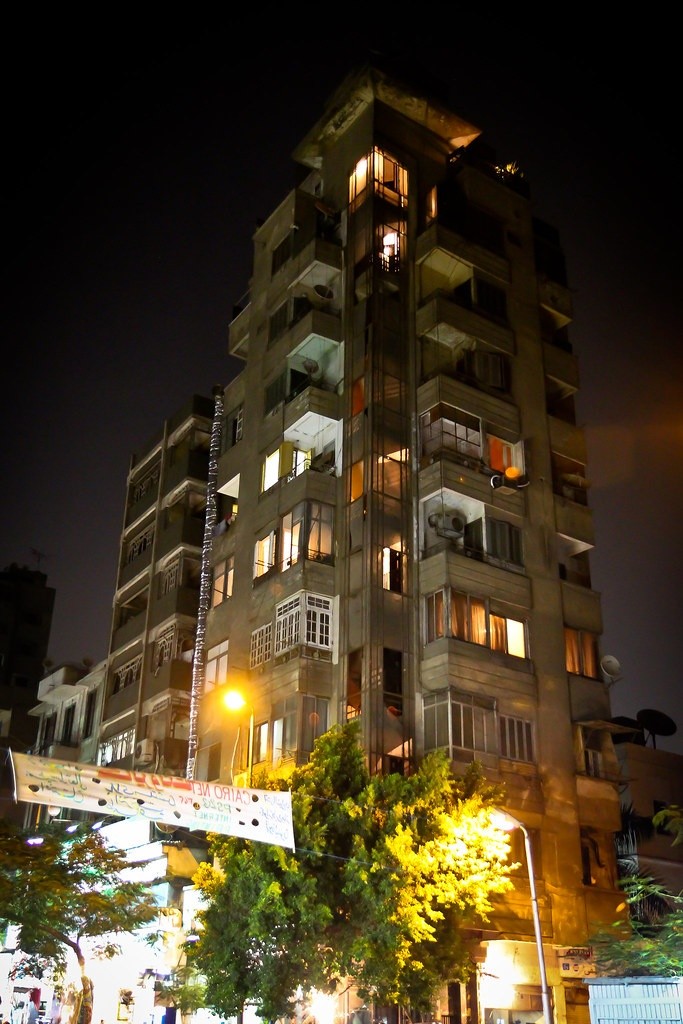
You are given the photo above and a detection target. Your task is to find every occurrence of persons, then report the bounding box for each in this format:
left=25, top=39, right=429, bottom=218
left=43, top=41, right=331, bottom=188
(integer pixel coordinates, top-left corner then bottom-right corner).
left=24, top=987, right=79, bottom=1024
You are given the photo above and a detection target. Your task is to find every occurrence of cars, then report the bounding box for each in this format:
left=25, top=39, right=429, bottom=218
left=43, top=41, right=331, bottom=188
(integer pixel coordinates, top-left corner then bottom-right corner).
left=14, top=1000, right=53, bottom=1024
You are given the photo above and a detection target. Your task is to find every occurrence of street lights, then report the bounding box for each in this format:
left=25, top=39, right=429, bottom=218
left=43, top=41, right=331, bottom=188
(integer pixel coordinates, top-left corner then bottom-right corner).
left=488, top=807, right=553, bottom=1024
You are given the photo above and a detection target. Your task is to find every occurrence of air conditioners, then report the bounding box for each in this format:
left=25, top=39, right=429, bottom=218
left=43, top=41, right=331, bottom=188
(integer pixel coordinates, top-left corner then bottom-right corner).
left=437, top=514, right=465, bottom=539
